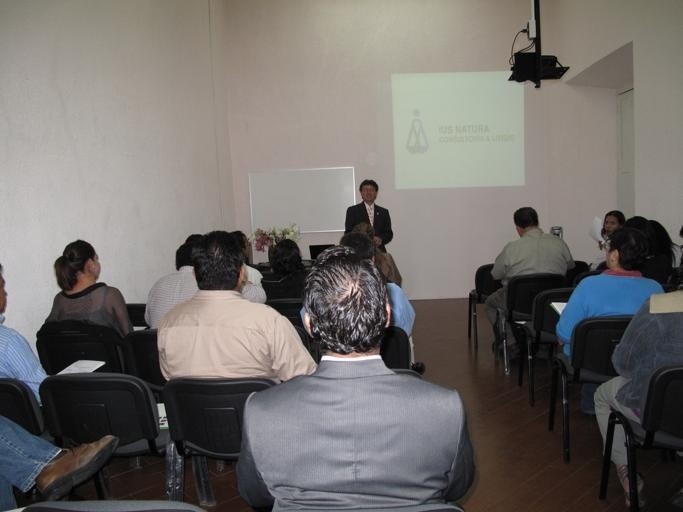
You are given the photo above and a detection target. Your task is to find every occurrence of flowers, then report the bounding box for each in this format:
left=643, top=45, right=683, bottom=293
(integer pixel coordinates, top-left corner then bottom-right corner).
left=251, top=220, right=302, bottom=253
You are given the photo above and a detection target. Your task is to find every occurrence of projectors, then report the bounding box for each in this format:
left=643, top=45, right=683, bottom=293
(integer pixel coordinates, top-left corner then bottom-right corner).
left=508, top=53, right=569, bottom=87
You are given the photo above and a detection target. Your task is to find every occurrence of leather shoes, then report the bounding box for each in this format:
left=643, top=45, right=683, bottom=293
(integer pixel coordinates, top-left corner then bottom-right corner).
left=34, top=435, right=119, bottom=501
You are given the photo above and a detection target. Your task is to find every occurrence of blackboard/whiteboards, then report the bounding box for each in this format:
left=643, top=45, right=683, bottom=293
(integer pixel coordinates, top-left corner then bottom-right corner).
left=248, top=166, right=356, bottom=234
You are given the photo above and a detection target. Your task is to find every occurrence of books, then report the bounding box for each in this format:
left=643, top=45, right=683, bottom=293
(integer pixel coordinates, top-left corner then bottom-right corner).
left=58, top=359, right=106, bottom=376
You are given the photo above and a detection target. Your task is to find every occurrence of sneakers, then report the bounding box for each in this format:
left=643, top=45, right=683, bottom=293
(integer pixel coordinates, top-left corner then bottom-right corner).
left=616, top=464, right=646, bottom=510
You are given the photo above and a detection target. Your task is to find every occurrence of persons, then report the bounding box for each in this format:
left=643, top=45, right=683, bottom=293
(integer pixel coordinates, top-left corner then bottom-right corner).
left=267, top=240, right=302, bottom=281
left=600, top=216, right=679, bottom=282
left=46, top=238, right=136, bottom=350
left=592, top=224, right=683, bottom=512
left=0, top=261, right=48, bottom=406
left=338, top=231, right=416, bottom=338
left=600, top=212, right=624, bottom=243
left=156, top=230, right=319, bottom=383
left=0, top=413, right=120, bottom=508
left=555, top=228, right=665, bottom=416
left=484, top=206, right=574, bottom=350
left=230, top=230, right=264, bottom=284
left=343, top=179, right=394, bottom=249
left=231, top=244, right=477, bottom=511
left=143, top=245, right=267, bottom=329
left=347, top=222, right=402, bottom=288
